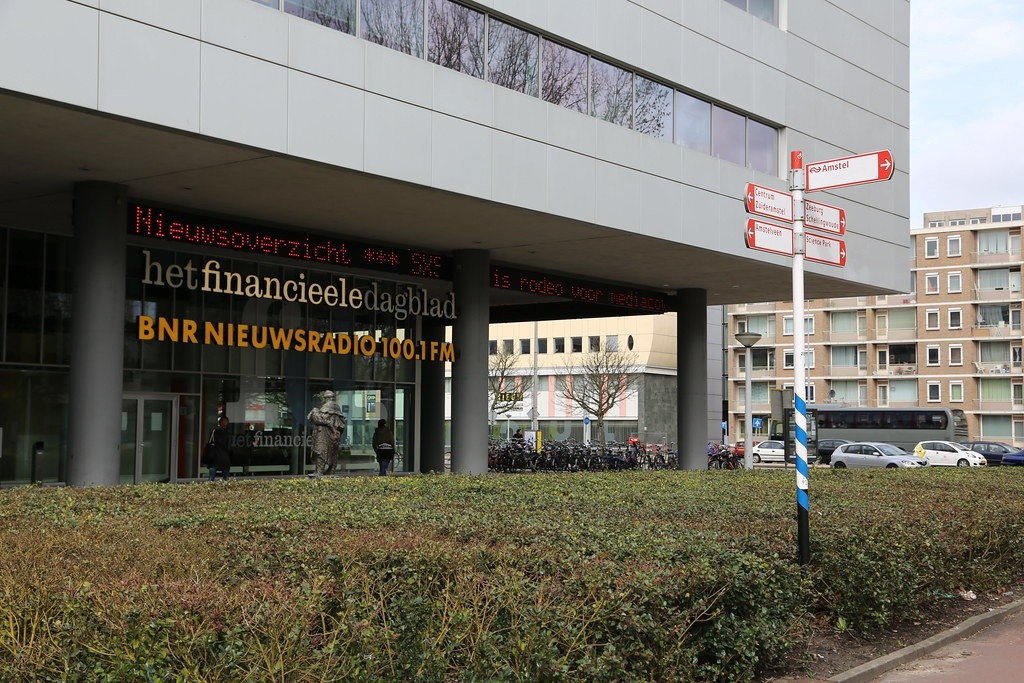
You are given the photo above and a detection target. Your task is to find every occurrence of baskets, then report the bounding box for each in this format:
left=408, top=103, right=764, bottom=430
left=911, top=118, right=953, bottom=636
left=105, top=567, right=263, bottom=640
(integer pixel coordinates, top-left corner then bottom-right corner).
left=721, top=451, right=730, bottom=458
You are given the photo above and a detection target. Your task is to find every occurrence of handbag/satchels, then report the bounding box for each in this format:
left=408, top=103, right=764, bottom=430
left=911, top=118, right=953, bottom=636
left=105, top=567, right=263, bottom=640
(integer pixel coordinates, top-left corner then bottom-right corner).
left=202, top=428, right=217, bottom=464
left=377, top=442, right=394, bottom=459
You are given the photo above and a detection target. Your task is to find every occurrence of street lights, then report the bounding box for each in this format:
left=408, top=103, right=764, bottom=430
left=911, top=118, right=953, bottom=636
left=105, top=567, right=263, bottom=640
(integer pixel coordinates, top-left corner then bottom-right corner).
left=734, top=332, right=764, bottom=469
left=504, top=414, right=512, bottom=441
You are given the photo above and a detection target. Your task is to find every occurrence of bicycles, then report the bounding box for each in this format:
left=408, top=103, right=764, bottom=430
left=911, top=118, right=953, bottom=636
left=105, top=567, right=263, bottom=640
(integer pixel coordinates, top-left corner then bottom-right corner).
left=444, top=434, right=747, bottom=472
left=394, top=440, right=404, bottom=467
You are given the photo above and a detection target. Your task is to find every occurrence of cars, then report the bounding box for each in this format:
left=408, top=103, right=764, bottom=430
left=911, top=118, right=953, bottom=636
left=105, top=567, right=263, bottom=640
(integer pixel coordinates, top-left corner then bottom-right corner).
left=732, top=441, right=760, bottom=458
left=1000, top=447, right=1024, bottom=466
left=752, top=440, right=785, bottom=463
left=817, top=439, right=852, bottom=464
left=959, top=439, right=1020, bottom=466
left=829, top=442, right=933, bottom=470
left=912, top=440, right=988, bottom=468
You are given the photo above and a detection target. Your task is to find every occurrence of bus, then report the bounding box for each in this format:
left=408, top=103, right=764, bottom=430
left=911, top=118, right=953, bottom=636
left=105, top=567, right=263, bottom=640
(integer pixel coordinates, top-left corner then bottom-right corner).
left=804, top=404, right=969, bottom=453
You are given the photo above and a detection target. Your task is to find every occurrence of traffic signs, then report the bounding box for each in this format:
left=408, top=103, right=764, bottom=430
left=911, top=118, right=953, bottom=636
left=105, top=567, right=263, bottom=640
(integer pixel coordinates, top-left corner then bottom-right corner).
left=803, top=233, right=849, bottom=271
left=744, top=217, right=794, bottom=256
left=804, top=150, right=895, bottom=196
left=747, top=183, right=795, bottom=222
left=803, top=197, right=848, bottom=234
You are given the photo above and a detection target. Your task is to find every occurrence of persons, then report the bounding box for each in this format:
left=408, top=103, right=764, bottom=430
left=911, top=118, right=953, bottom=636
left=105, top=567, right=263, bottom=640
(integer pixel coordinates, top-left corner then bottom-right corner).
left=207, top=416, right=233, bottom=481
left=902, top=361, right=908, bottom=375
left=307, top=390, right=346, bottom=478
left=975, top=445, right=1003, bottom=452
left=372, top=419, right=395, bottom=476
left=512, top=429, right=523, bottom=442
